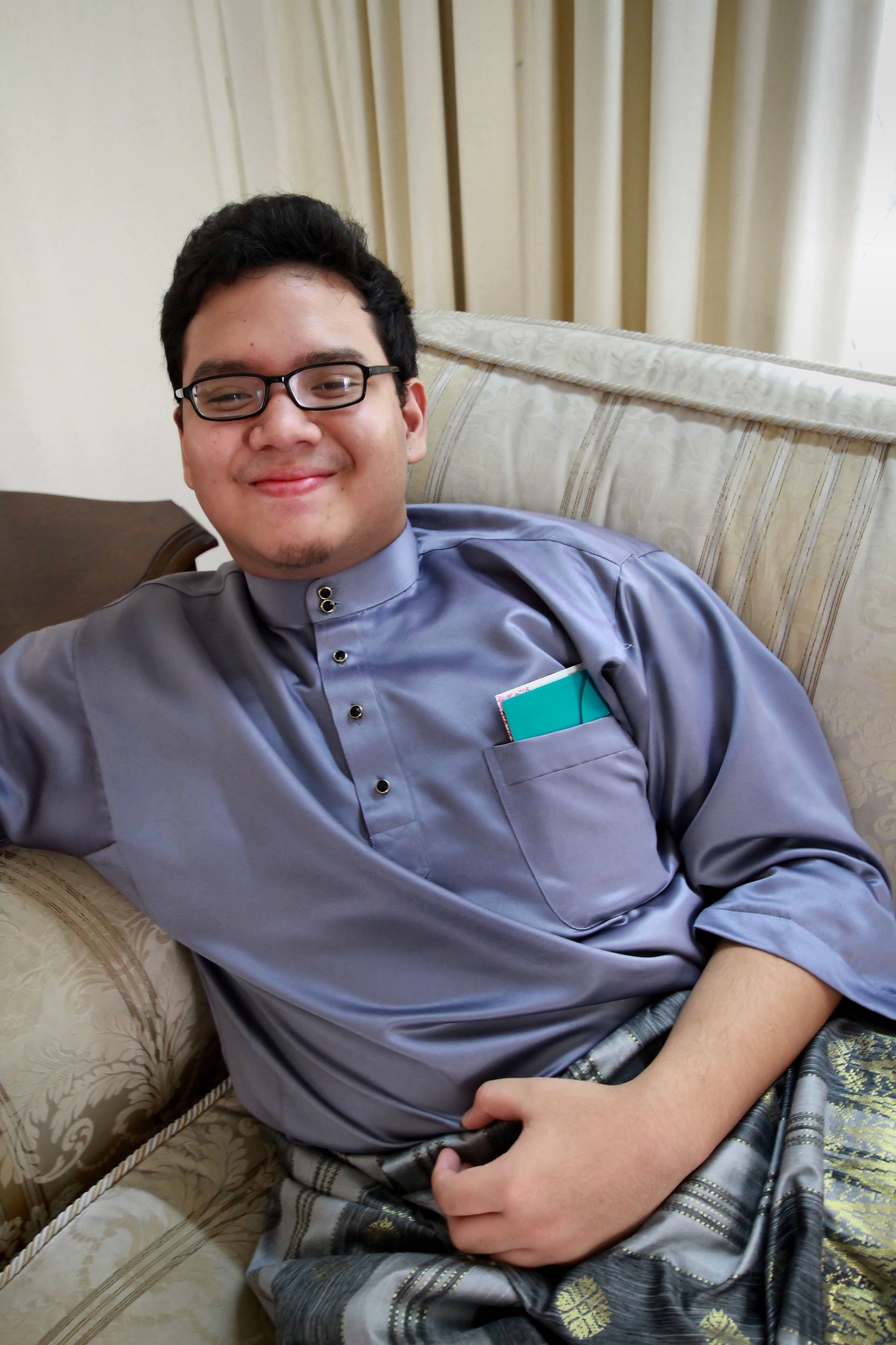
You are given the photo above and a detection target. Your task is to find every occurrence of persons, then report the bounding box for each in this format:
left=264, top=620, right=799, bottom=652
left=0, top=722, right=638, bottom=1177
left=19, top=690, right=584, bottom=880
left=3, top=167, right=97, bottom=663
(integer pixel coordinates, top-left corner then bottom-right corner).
left=1, top=190, right=896, bottom=1345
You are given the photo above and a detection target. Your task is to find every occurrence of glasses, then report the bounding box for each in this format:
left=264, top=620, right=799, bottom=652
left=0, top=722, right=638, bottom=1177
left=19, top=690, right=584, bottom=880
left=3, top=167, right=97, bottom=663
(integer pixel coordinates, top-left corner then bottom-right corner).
left=176, top=361, right=400, bottom=423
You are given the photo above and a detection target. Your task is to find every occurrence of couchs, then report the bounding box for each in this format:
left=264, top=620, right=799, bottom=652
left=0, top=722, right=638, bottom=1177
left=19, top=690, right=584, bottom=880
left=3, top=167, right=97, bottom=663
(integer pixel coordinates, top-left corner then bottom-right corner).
left=0, top=306, right=896, bottom=1345
